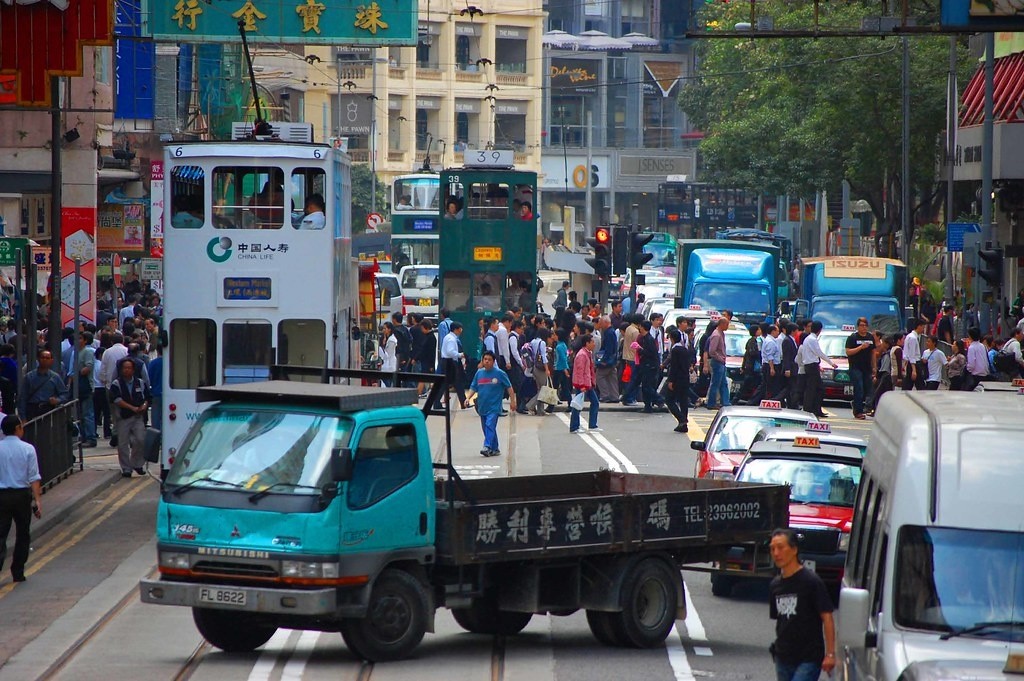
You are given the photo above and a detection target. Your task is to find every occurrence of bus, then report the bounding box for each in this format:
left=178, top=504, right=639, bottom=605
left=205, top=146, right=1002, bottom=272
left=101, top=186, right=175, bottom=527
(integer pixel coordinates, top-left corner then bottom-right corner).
left=389, top=131, right=441, bottom=280
left=654, top=170, right=775, bottom=241
left=142, top=14, right=360, bottom=493
left=436, top=140, right=540, bottom=392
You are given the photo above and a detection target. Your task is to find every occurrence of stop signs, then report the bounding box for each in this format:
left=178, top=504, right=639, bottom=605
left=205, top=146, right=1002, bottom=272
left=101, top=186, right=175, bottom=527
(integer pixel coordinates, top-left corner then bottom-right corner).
left=113, top=252, right=122, bottom=289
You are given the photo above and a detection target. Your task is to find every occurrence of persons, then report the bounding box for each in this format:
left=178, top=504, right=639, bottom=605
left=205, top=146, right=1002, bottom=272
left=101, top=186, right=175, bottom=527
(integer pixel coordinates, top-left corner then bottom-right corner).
left=173, top=194, right=234, bottom=228
left=0, top=276, right=162, bottom=478
left=446, top=196, right=463, bottom=219
left=466, top=59, right=476, bottom=71
left=435, top=307, right=454, bottom=373
left=481, top=283, right=491, bottom=295
left=397, top=195, right=411, bottom=209
left=300, top=194, right=325, bottom=230
left=261, top=168, right=294, bottom=229
left=389, top=56, right=397, bottom=66
left=457, top=140, right=468, bottom=151
left=480, top=290, right=733, bottom=433
left=769, top=528, right=836, bottom=681
left=557, top=281, right=568, bottom=311
left=0, top=416, right=41, bottom=581
left=735, top=319, right=837, bottom=416
left=513, top=200, right=532, bottom=220
left=541, top=238, right=563, bottom=270
left=845, top=282, right=1024, bottom=418
left=435, top=322, right=474, bottom=410
left=464, top=350, right=515, bottom=457
left=379, top=312, right=436, bottom=396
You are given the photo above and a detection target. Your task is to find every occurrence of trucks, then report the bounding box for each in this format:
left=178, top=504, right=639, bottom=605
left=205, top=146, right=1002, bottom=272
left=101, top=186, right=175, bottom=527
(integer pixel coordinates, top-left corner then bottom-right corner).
left=660, top=238, right=782, bottom=336
left=136, top=360, right=795, bottom=663
left=788, top=254, right=908, bottom=351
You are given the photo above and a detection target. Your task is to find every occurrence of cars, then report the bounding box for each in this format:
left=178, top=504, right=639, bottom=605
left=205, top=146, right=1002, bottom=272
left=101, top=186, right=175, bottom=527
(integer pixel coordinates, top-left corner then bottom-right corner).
left=730, top=420, right=872, bottom=476
left=710, top=436, right=866, bottom=612
left=598, top=265, right=883, bottom=404
left=689, top=399, right=821, bottom=481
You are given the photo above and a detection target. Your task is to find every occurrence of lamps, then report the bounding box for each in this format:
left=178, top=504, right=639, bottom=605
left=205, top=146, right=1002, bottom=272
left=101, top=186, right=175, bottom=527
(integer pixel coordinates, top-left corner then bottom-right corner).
left=112, top=149, right=136, bottom=161
left=62, top=127, right=81, bottom=143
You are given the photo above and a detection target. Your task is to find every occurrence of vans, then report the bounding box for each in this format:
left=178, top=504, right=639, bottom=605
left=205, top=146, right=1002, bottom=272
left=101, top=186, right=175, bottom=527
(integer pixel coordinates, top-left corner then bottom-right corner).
left=371, top=262, right=406, bottom=331
left=397, top=264, right=440, bottom=329
left=823, top=387, right=1024, bottom=681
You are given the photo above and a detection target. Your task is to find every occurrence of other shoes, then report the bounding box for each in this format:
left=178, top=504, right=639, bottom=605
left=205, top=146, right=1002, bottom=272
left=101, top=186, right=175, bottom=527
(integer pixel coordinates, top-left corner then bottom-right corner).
left=708, top=405, right=720, bottom=410
left=134, top=466, right=146, bottom=475
left=13, top=576, right=26, bottom=582
left=589, top=427, right=604, bottom=432
left=81, top=441, right=96, bottom=448
left=816, top=413, right=828, bottom=418
left=104, top=435, right=111, bottom=438
left=480, top=447, right=491, bottom=456
left=694, top=399, right=703, bottom=409
left=856, top=413, right=865, bottom=419
left=109, top=435, right=119, bottom=447
left=600, top=397, right=665, bottom=413
left=498, top=407, right=551, bottom=416
left=490, top=449, right=500, bottom=456
left=571, top=428, right=586, bottom=434
left=122, top=471, right=132, bottom=477
left=418, top=394, right=427, bottom=399
left=674, top=423, right=688, bottom=432
left=688, top=402, right=695, bottom=408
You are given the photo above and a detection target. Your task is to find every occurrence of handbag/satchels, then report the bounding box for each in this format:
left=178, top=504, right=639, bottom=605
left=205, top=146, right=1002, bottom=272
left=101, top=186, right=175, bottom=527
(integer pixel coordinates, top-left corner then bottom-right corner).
left=535, top=340, right=554, bottom=370
left=921, top=361, right=929, bottom=380
left=570, top=392, right=585, bottom=411
left=537, top=375, right=558, bottom=406
left=689, top=371, right=697, bottom=383
left=621, top=363, right=632, bottom=383
left=993, top=351, right=1016, bottom=371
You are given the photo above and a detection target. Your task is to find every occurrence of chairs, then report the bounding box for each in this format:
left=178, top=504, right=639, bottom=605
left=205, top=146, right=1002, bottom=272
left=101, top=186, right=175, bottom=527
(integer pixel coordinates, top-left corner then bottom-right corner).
left=362, top=426, right=417, bottom=503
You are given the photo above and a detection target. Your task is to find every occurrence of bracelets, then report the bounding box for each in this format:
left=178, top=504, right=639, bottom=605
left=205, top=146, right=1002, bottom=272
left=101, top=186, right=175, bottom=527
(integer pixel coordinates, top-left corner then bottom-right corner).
left=826, top=653, right=834, bottom=657
left=144, top=403, right=146, bottom=405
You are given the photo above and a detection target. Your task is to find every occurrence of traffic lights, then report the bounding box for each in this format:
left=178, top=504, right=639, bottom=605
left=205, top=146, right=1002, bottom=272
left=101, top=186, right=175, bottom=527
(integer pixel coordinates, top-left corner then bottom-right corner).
left=629, top=231, right=654, bottom=269
left=584, top=237, right=596, bottom=270
left=977, top=247, right=1004, bottom=287
left=594, top=225, right=612, bottom=275
